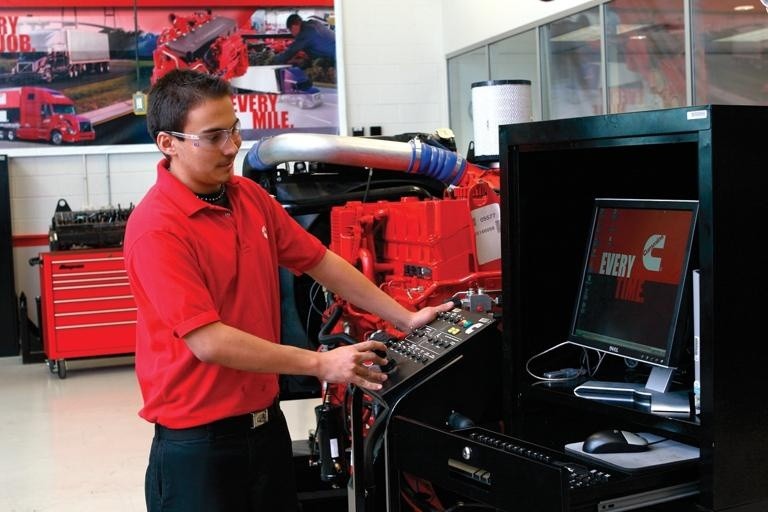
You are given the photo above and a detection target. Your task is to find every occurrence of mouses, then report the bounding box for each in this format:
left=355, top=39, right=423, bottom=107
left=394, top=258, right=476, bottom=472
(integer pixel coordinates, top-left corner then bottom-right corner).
left=582, top=429, right=649, bottom=454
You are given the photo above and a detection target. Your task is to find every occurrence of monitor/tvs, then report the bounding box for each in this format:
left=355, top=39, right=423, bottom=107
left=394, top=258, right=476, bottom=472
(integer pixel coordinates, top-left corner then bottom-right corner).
left=568, top=196, right=700, bottom=413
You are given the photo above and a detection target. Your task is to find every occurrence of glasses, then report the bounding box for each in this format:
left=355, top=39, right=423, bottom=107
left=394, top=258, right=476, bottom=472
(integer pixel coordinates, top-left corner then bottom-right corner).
left=155, top=118, right=241, bottom=150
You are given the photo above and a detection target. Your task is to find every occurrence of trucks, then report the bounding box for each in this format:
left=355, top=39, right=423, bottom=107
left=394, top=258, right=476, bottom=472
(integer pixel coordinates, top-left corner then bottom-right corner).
left=0, top=85, right=96, bottom=145
left=10, top=27, right=111, bottom=85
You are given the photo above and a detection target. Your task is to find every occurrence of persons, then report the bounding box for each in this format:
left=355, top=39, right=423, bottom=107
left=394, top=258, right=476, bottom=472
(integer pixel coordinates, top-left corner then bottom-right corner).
left=121, top=67, right=457, bottom=511
left=259, top=15, right=335, bottom=71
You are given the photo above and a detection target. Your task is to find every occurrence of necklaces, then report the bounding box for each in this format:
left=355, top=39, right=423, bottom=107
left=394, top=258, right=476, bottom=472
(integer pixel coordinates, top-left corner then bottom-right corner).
left=193, top=184, right=227, bottom=202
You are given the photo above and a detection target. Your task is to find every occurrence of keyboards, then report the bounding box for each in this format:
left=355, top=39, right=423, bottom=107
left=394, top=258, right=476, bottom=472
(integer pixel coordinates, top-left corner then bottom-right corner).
left=460, top=432, right=613, bottom=506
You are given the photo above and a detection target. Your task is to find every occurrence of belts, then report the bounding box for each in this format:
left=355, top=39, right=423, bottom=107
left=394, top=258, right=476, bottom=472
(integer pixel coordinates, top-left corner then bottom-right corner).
left=159, top=402, right=280, bottom=442
